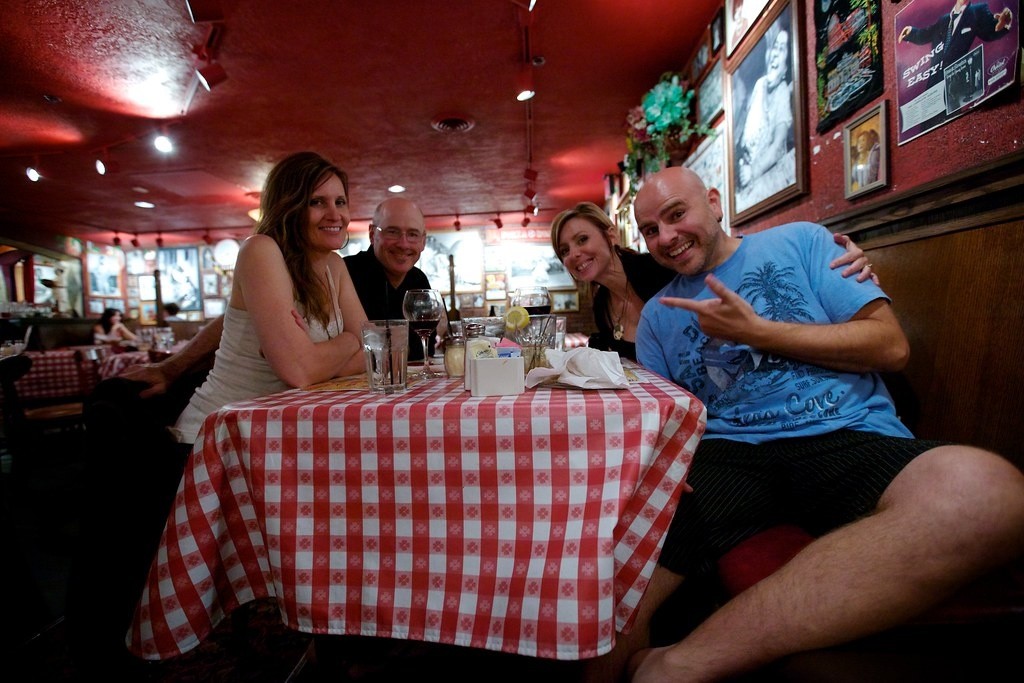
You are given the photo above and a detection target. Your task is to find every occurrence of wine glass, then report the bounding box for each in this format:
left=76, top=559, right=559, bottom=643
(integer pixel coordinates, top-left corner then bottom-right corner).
left=402, top=289, right=445, bottom=381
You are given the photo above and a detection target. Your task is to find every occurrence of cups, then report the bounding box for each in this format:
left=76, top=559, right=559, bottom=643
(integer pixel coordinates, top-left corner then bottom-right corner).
left=512, top=314, right=566, bottom=382
left=359, top=319, right=409, bottom=395
left=136, top=327, right=174, bottom=354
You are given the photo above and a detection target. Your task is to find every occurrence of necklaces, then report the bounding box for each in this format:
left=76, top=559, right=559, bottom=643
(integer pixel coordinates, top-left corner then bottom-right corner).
left=308, top=271, right=340, bottom=340
left=609, top=276, right=629, bottom=341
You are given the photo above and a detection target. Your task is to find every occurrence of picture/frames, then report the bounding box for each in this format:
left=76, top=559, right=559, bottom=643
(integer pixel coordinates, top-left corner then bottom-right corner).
left=696, top=55, right=724, bottom=138
left=724, top=1, right=810, bottom=227
left=710, top=7, right=724, bottom=58
left=843, top=99, right=890, bottom=200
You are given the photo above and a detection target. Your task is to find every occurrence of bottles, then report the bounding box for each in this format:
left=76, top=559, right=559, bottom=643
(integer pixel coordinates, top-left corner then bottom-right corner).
left=444, top=321, right=487, bottom=379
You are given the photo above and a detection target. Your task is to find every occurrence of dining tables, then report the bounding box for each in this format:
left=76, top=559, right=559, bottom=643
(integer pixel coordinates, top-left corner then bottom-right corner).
left=13, top=331, right=708, bottom=662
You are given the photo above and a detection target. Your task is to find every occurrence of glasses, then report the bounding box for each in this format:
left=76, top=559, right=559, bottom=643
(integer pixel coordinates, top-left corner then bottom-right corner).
left=375, top=226, right=425, bottom=243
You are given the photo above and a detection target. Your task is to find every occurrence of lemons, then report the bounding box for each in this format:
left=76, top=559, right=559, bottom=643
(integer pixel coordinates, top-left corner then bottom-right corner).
left=503, top=306, right=530, bottom=331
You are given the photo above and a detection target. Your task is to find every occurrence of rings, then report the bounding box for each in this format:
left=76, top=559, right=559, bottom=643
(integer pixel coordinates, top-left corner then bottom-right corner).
left=862, top=264, right=873, bottom=268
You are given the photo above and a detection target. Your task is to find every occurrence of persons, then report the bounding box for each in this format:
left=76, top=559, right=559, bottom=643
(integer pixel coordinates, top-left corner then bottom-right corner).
left=734, top=22, right=792, bottom=189
left=166, top=152, right=384, bottom=472
left=586, top=168, right=1024, bottom=683
left=163, top=303, right=185, bottom=322
left=851, top=131, right=880, bottom=189
left=552, top=201, right=881, bottom=361
left=899, top=0, right=1013, bottom=132
left=94, top=307, right=136, bottom=345
left=117, top=195, right=437, bottom=398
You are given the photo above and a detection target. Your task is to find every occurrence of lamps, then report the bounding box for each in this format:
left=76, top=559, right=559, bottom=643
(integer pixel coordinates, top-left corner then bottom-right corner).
left=113, top=230, right=163, bottom=247
left=190, top=43, right=228, bottom=91
left=202, top=228, right=212, bottom=244
left=514, top=67, right=535, bottom=102
left=495, top=213, right=503, bottom=228
left=455, top=214, right=460, bottom=231
left=522, top=169, right=538, bottom=228
left=512, top=0, right=537, bottom=12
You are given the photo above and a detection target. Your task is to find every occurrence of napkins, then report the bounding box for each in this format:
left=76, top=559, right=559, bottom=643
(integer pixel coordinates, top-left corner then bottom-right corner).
left=527, top=348, right=630, bottom=392
